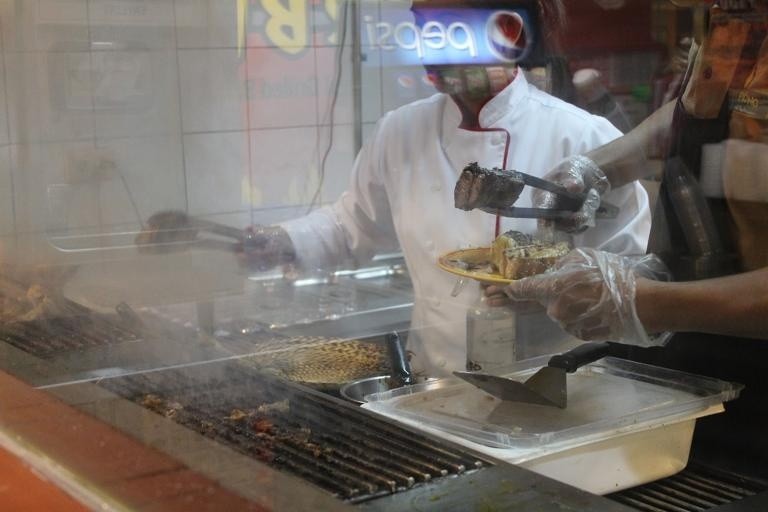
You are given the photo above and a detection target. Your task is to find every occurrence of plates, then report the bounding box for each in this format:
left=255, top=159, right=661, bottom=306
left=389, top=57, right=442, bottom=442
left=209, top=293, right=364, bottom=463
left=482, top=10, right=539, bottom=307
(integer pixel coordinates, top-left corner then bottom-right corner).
left=436, top=247, right=515, bottom=287
left=249, top=334, right=392, bottom=390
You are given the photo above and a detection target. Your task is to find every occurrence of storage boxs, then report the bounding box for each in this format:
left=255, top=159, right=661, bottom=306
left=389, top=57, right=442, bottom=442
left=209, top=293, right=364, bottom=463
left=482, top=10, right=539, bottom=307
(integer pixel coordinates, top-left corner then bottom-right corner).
left=358, top=347, right=748, bottom=497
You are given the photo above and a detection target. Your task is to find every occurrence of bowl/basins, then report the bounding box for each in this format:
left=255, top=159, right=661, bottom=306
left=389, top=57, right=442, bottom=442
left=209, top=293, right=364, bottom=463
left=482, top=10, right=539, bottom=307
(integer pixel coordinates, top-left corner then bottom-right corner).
left=341, top=373, right=441, bottom=405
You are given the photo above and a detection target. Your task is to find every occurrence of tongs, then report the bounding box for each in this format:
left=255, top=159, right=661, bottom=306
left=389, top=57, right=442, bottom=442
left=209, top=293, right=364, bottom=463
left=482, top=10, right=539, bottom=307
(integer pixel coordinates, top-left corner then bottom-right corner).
left=479, top=167, right=620, bottom=220
left=186, top=217, right=262, bottom=253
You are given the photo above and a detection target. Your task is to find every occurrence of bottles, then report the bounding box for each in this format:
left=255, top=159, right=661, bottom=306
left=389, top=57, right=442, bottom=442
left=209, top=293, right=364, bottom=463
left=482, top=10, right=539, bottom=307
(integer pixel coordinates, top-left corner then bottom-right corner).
left=664, top=156, right=722, bottom=259
left=573, top=68, right=654, bottom=183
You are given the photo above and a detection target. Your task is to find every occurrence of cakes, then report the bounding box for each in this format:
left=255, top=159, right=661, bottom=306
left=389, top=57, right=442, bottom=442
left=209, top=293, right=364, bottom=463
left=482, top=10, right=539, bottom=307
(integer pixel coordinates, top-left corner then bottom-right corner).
left=499, top=244, right=569, bottom=280
left=488, top=231, right=532, bottom=274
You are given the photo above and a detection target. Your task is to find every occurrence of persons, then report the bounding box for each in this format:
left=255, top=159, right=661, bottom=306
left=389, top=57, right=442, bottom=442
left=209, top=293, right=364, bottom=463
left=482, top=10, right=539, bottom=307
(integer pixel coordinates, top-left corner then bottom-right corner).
left=233, top=0, right=655, bottom=393
left=501, top=0, right=766, bottom=466
